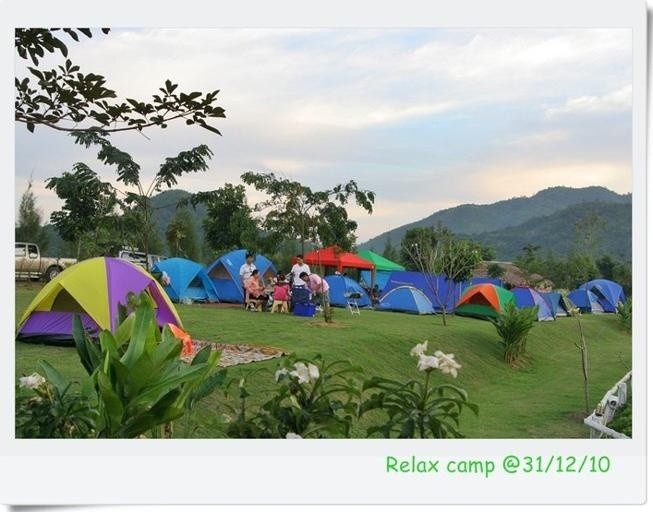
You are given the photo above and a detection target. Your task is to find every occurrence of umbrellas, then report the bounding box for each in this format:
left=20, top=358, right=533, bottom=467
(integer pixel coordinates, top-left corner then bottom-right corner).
left=349, top=249, right=405, bottom=275
left=291, top=244, right=375, bottom=274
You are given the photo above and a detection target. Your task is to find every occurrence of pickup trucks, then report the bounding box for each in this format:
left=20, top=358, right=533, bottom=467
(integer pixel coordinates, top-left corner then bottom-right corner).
left=15, top=242, right=80, bottom=285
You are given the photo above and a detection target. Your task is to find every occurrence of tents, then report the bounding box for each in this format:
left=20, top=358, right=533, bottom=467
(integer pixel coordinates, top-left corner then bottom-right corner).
left=195, top=249, right=278, bottom=303
left=14, top=255, right=191, bottom=356
left=321, top=272, right=627, bottom=322
left=150, top=255, right=220, bottom=304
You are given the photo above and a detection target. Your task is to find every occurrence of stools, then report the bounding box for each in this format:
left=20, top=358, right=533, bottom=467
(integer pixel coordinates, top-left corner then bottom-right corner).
left=270, top=300, right=288, bottom=313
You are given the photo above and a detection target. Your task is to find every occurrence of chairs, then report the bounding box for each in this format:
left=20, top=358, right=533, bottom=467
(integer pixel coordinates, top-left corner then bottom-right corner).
left=245, top=291, right=267, bottom=312
left=343, top=292, right=363, bottom=316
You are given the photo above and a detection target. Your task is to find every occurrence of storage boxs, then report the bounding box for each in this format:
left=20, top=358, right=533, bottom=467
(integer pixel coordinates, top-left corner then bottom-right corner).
left=292, top=302, right=317, bottom=317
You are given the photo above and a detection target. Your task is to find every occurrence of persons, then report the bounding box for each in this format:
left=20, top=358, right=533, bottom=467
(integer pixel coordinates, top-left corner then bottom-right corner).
left=239, top=251, right=330, bottom=315
